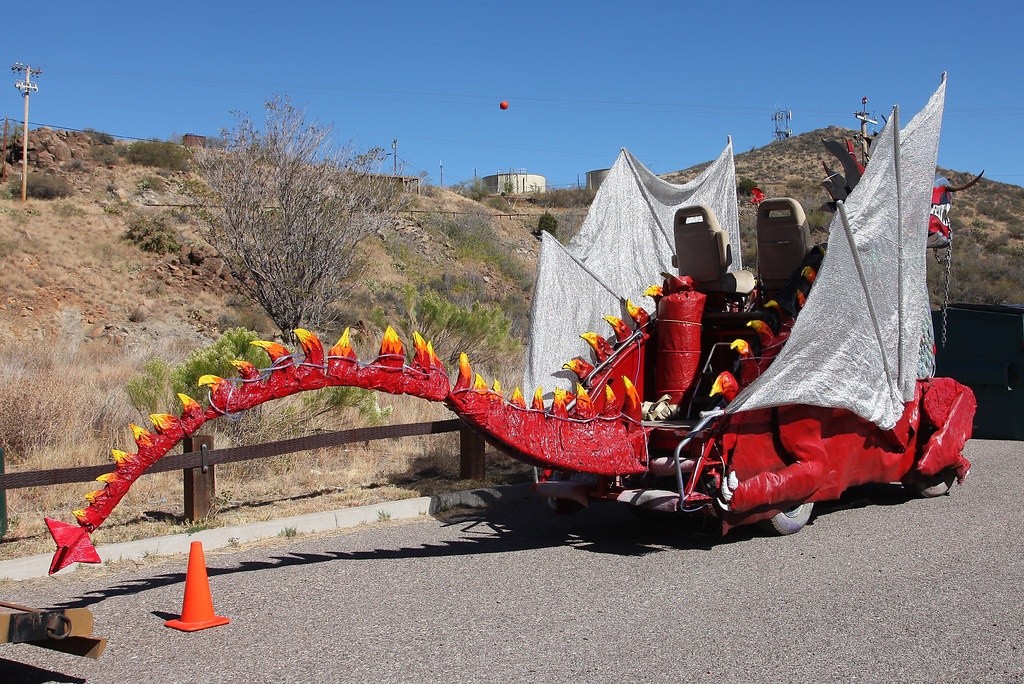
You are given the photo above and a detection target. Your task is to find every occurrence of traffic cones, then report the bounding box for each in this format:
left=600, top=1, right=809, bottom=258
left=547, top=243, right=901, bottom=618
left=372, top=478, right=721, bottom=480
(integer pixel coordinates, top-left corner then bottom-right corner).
left=163, top=542, right=230, bottom=633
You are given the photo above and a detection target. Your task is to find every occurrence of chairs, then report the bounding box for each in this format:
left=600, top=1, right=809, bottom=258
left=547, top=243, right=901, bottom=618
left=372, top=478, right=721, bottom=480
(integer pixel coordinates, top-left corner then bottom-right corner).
left=755, top=197, right=827, bottom=303
left=670, top=204, right=755, bottom=312
